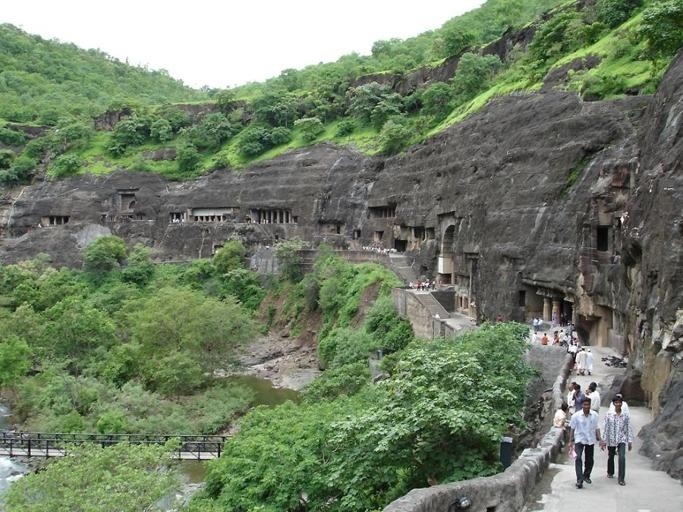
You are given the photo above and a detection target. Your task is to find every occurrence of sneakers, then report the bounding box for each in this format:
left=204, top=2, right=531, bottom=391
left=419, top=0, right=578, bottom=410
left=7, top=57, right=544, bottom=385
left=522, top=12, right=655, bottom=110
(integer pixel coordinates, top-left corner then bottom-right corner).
left=605, top=473, right=626, bottom=486
left=574, top=475, right=591, bottom=489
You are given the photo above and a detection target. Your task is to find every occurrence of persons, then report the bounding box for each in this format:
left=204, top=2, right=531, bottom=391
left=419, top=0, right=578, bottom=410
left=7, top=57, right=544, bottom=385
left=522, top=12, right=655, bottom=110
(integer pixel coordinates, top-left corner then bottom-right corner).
left=362, top=247, right=397, bottom=254
left=260, top=218, right=269, bottom=224
left=600, top=396, right=632, bottom=486
left=553, top=382, right=600, bottom=442
left=253, top=220, right=256, bottom=224
left=609, top=393, right=631, bottom=455
left=477, top=312, right=488, bottom=324
left=248, top=219, right=250, bottom=223
left=496, top=315, right=502, bottom=322
left=172, top=218, right=184, bottom=223
left=530, top=311, right=593, bottom=376
left=569, top=397, right=601, bottom=488
left=410, top=277, right=435, bottom=291
